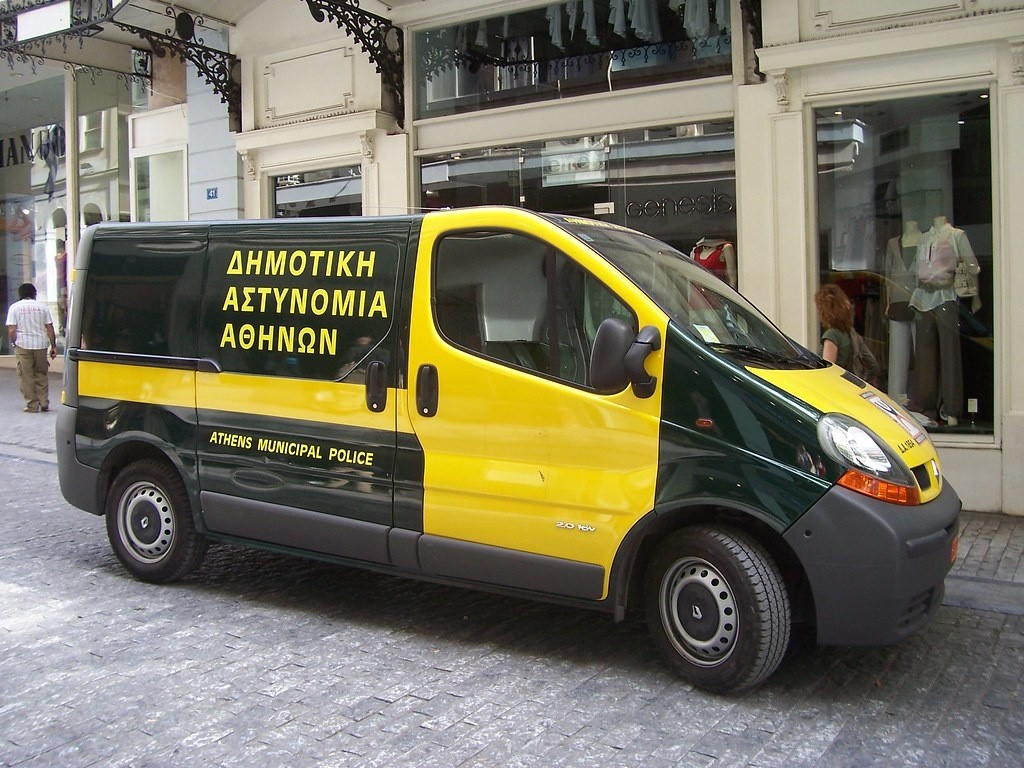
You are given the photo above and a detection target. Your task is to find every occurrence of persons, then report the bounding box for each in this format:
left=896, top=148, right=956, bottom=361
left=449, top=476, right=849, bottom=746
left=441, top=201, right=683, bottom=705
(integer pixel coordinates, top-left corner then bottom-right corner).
left=55, top=240, right=67, bottom=333
left=687, top=238, right=737, bottom=324
left=907, top=216, right=982, bottom=427
left=5, top=283, right=57, bottom=412
left=815, top=284, right=878, bottom=383
left=885, top=222, right=926, bottom=400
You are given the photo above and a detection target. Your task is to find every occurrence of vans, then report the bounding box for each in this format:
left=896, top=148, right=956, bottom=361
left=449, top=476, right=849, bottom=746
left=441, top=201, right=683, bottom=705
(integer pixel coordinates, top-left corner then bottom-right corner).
left=709, top=265, right=993, bottom=435
left=55, top=204, right=964, bottom=697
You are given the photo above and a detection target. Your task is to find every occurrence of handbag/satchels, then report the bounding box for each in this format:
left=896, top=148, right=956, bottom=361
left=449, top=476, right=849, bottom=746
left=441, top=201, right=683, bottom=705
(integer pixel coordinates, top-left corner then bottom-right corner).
left=847, top=327, right=879, bottom=384
left=953, top=261, right=978, bottom=297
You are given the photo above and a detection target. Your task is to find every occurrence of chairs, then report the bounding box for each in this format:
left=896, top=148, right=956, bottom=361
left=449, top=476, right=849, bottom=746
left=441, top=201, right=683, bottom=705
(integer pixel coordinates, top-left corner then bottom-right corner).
left=538, top=296, right=598, bottom=389
